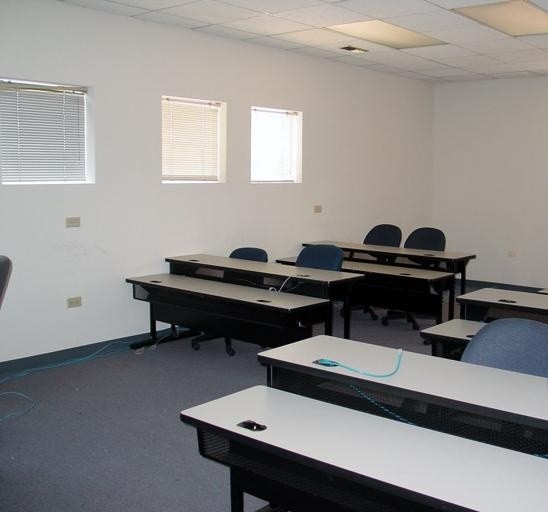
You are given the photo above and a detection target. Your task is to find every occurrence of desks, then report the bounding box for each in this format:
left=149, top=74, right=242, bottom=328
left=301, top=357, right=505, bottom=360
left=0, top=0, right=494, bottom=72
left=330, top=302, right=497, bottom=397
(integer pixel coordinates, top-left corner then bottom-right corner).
left=456, top=287, right=547, bottom=321
left=302, top=240, right=477, bottom=320
left=257, top=335, right=546, bottom=430
left=275, top=256, right=455, bottom=345
left=180, top=385, right=546, bottom=510
left=126, top=273, right=333, bottom=364
left=158, top=254, right=365, bottom=350
left=420, top=320, right=489, bottom=357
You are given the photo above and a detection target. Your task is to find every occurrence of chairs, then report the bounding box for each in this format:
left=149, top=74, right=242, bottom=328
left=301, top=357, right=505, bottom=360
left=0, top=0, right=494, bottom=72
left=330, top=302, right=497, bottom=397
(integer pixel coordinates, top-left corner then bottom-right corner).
left=340, top=224, right=402, bottom=320
left=440, top=318, right=546, bottom=454
left=243, top=244, right=343, bottom=347
left=192, top=247, right=268, bottom=351
left=0, top=254, right=11, bottom=306
left=382, top=227, right=446, bottom=330
